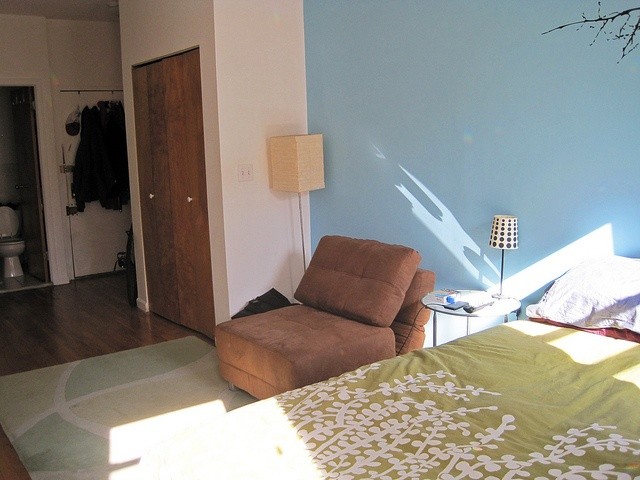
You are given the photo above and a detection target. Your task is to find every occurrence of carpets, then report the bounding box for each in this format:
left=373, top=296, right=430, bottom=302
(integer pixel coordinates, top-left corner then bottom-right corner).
left=1, top=333, right=261, bottom=480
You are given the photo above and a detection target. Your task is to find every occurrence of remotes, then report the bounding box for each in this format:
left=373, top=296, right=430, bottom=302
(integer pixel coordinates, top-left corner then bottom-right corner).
left=463, top=298, right=494, bottom=312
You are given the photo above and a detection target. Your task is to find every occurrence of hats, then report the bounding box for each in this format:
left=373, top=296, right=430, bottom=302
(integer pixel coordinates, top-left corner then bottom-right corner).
left=65, top=110, right=80, bottom=136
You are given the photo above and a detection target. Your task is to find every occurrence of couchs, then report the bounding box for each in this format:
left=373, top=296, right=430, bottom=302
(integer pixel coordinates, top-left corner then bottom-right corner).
left=215, top=236, right=435, bottom=400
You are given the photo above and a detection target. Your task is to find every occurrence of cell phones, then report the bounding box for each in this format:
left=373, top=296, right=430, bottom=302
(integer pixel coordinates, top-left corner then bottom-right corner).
left=443, top=300, right=468, bottom=310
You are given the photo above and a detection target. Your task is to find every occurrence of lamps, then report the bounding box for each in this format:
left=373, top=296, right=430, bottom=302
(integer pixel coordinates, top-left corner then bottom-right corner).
left=489, top=216, right=518, bottom=298
left=268, top=134, right=325, bottom=273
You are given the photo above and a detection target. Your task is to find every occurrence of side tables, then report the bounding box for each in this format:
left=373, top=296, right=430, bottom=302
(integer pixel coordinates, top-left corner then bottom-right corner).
left=421, top=290, right=522, bottom=347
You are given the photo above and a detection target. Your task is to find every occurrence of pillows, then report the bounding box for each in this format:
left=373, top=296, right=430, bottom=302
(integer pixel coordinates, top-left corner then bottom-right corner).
left=527, top=252, right=639, bottom=342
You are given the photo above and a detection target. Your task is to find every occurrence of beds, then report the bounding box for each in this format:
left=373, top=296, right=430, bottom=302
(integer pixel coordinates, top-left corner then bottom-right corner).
left=110, top=319, right=639, bottom=480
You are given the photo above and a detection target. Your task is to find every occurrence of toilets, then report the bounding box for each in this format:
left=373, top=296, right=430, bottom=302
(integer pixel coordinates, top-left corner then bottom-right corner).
left=0, top=206, right=26, bottom=278
